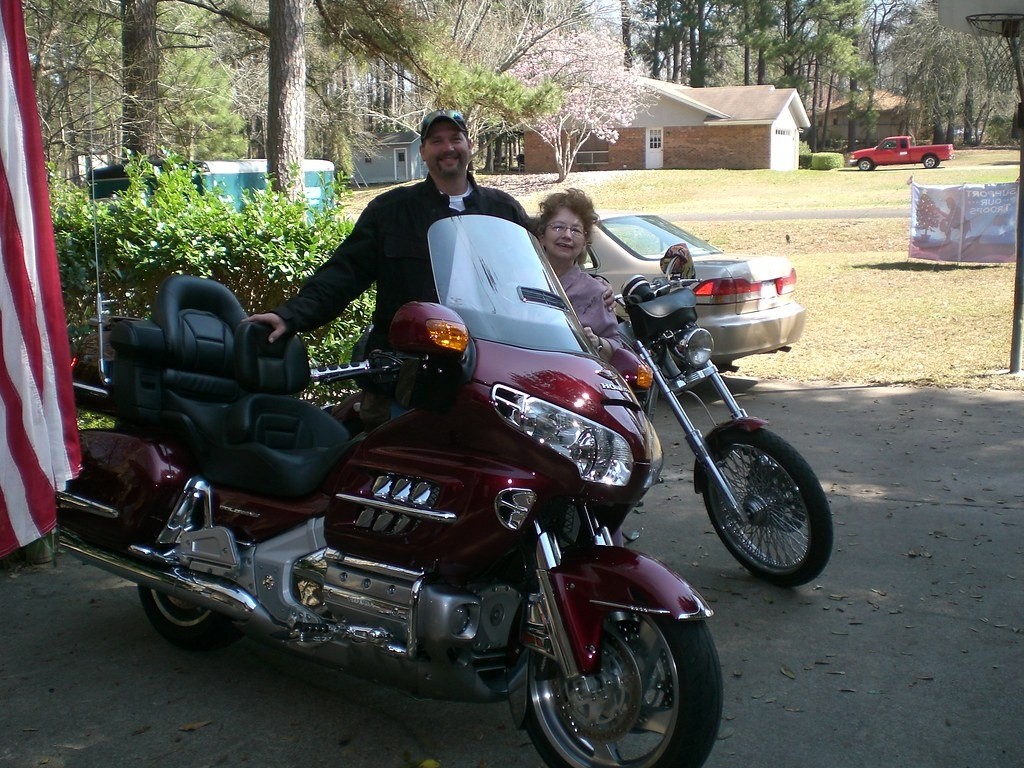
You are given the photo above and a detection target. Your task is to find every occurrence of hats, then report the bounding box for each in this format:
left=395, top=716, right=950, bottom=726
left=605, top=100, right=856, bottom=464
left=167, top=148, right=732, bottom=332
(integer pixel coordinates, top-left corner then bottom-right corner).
left=419, top=110, right=475, bottom=141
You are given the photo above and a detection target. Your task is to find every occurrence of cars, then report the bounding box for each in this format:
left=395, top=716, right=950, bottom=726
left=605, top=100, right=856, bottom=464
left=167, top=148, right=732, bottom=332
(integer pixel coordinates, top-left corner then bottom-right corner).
left=523, top=209, right=806, bottom=374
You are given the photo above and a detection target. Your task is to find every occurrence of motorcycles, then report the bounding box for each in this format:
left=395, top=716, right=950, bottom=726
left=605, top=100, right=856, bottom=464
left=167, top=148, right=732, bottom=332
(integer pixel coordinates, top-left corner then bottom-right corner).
left=54, top=211, right=836, bottom=768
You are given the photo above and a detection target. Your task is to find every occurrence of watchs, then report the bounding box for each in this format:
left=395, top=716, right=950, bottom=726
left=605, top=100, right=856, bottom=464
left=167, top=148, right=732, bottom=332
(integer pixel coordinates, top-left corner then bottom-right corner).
left=596, top=336, right=603, bottom=352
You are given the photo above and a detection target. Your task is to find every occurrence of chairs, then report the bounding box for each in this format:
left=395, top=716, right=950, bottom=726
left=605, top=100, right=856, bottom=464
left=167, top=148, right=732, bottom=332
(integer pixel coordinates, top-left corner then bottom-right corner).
left=108, top=271, right=250, bottom=487
left=226, top=319, right=354, bottom=502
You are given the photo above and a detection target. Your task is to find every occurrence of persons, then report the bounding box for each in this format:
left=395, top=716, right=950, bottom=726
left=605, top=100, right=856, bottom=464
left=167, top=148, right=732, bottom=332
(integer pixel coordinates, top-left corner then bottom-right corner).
left=528, top=187, right=623, bottom=362
left=242, top=109, right=617, bottom=392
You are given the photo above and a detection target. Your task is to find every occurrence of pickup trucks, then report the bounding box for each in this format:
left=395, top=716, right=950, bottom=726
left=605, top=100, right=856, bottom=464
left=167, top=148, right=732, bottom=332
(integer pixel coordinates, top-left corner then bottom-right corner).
left=848, top=136, right=955, bottom=172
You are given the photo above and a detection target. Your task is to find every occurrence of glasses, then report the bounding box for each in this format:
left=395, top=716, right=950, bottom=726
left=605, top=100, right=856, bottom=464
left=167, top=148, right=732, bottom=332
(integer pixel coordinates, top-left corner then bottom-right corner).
left=544, top=219, right=591, bottom=244
